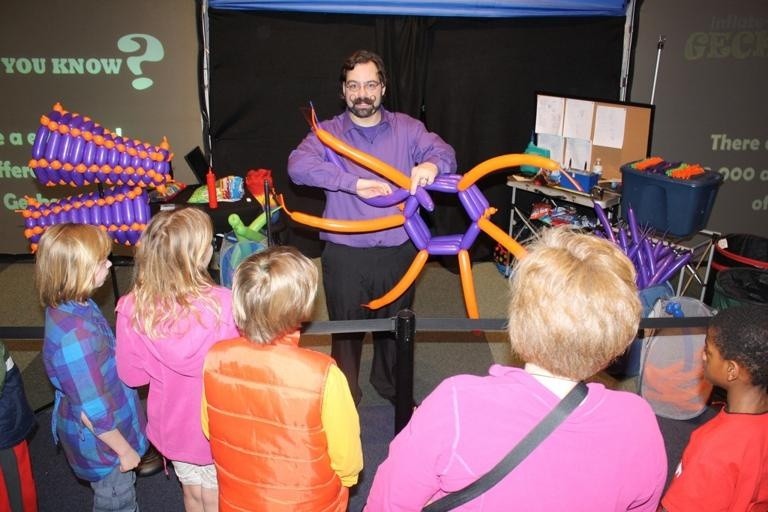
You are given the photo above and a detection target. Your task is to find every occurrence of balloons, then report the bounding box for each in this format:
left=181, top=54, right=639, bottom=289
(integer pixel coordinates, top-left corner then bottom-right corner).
left=588, top=201, right=691, bottom=288
left=665, top=300, right=694, bottom=374
left=236, top=224, right=268, bottom=245
left=231, top=208, right=283, bottom=264
left=16, top=101, right=168, bottom=247
left=226, top=207, right=273, bottom=246
left=276, top=112, right=582, bottom=318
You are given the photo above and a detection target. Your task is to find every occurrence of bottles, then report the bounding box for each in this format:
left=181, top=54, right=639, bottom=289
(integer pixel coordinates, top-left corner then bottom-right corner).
left=205, top=166, right=220, bottom=209
left=591, top=158, right=604, bottom=179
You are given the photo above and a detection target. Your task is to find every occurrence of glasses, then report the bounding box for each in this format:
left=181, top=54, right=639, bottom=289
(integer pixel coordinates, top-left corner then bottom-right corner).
left=344, top=81, right=382, bottom=92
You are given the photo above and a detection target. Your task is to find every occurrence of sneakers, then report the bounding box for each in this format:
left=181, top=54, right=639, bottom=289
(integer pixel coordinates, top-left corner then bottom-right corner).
left=378, top=391, right=417, bottom=412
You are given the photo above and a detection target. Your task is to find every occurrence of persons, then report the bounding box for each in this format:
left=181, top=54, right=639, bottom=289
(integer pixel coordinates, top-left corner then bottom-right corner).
left=31, top=220, right=151, bottom=512
left=1, top=341, right=42, bottom=512
left=284, top=47, right=459, bottom=410
left=197, top=245, right=365, bottom=512
left=349, top=221, right=669, bottom=512
left=656, top=302, right=767, bottom=512
left=112, top=205, right=247, bottom=512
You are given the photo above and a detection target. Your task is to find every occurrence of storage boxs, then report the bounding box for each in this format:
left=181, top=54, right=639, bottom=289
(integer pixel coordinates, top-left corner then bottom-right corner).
left=620, top=159, right=724, bottom=236
